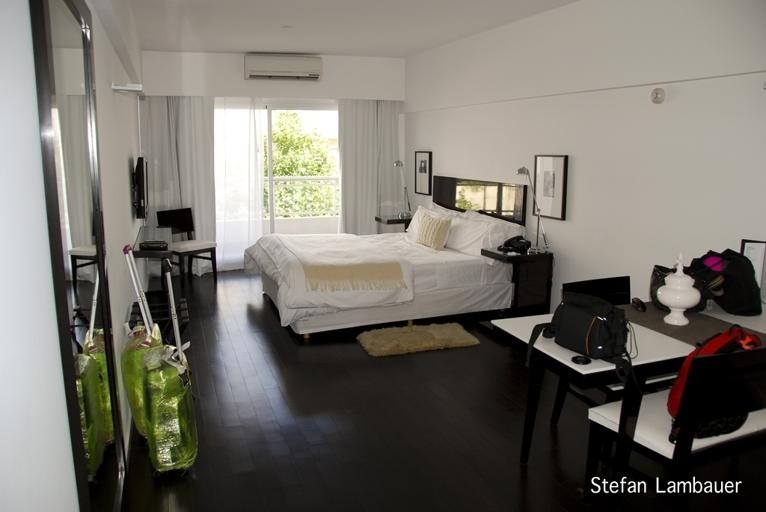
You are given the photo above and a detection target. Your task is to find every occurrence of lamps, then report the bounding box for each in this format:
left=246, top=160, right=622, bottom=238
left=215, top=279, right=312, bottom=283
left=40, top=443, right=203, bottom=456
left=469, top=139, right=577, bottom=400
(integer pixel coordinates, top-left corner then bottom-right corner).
left=394, top=160, right=412, bottom=220
left=515, top=161, right=551, bottom=253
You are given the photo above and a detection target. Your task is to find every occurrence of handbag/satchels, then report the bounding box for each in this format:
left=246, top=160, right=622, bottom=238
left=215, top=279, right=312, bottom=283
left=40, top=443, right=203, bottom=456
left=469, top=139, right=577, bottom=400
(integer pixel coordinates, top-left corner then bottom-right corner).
left=523, top=290, right=633, bottom=383
left=666, top=322, right=763, bottom=447
left=650, top=249, right=763, bottom=318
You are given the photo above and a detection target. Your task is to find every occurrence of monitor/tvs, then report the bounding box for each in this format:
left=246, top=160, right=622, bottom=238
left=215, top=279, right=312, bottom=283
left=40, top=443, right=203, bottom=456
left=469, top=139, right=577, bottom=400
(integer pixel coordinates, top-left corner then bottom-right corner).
left=133, top=156, right=148, bottom=218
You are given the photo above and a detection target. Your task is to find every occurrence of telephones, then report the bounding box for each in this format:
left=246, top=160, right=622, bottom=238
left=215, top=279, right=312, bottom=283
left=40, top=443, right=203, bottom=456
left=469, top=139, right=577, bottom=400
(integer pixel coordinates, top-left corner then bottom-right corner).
left=504, top=236, right=531, bottom=253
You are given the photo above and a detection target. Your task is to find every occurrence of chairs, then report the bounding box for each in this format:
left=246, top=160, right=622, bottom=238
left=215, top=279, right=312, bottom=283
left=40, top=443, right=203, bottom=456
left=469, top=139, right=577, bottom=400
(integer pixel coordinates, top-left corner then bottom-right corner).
left=157, top=208, right=218, bottom=281
left=551, top=273, right=632, bottom=434
left=68, top=214, right=107, bottom=306
left=588, top=347, right=766, bottom=485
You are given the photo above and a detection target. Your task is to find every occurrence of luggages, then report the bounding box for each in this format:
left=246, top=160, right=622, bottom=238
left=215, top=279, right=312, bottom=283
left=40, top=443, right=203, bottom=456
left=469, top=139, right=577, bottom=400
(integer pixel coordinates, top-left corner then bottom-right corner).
left=117, top=243, right=200, bottom=479
left=71, top=265, right=117, bottom=485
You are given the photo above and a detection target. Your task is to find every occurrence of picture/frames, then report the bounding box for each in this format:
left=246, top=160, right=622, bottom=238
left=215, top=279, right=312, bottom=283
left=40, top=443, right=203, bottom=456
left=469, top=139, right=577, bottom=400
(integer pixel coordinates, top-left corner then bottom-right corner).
left=414, top=152, right=432, bottom=196
left=535, top=156, right=565, bottom=218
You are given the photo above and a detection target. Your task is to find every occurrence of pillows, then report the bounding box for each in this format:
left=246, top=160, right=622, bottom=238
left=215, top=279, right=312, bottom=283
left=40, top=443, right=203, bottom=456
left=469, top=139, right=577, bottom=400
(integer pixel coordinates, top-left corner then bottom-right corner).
left=406, top=203, right=524, bottom=258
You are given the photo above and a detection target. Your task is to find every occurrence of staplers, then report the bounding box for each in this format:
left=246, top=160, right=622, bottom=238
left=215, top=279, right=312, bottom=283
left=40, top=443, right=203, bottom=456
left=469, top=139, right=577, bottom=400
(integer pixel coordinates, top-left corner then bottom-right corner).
left=632, top=297, right=647, bottom=312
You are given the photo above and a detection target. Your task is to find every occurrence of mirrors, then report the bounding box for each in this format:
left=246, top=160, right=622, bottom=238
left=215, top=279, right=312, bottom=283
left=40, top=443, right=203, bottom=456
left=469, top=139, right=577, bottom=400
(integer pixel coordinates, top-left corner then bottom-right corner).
left=27, top=2, right=128, bottom=511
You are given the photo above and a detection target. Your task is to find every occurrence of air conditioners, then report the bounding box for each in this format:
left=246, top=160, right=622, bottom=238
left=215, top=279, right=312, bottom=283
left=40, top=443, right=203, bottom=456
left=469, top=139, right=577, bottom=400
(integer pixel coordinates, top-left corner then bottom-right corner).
left=245, top=53, right=321, bottom=81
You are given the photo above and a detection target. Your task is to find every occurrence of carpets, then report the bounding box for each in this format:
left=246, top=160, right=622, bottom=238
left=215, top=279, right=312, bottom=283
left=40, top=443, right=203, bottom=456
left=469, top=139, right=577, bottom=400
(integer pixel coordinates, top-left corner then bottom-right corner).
left=357, top=323, right=480, bottom=357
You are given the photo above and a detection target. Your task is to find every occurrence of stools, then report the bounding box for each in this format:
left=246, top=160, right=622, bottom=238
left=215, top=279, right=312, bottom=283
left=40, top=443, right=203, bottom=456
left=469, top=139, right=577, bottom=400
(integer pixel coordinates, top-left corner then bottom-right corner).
left=129, top=299, right=189, bottom=346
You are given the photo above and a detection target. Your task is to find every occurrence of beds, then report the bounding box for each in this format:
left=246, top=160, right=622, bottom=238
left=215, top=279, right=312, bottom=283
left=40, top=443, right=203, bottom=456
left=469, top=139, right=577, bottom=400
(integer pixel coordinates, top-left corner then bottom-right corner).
left=244, top=176, right=528, bottom=340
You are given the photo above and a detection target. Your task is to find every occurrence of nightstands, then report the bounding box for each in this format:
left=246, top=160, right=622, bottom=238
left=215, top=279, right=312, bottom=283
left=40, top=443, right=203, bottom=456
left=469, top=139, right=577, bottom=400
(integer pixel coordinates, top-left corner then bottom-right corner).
left=375, top=216, right=412, bottom=232
left=480, top=248, right=553, bottom=312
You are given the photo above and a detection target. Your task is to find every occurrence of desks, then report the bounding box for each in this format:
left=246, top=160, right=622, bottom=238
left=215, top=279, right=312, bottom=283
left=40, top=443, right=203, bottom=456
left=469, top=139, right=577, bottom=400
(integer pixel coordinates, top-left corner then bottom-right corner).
left=490, top=298, right=766, bottom=485
